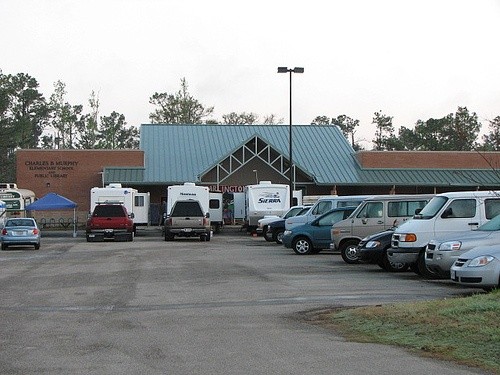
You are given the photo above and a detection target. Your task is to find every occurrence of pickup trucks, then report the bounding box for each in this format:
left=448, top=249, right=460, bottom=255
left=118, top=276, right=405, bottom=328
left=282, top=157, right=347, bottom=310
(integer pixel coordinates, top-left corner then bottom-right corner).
left=162, top=200, right=211, bottom=242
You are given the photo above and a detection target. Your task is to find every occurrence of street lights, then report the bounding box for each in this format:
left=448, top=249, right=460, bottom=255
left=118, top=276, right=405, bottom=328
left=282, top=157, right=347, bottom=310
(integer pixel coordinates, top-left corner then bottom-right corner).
left=276, top=66, right=304, bottom=209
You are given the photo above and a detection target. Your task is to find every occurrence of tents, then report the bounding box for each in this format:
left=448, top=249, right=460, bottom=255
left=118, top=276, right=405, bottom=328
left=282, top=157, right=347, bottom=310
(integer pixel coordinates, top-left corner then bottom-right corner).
left=25, top=193, right=78, bottom=237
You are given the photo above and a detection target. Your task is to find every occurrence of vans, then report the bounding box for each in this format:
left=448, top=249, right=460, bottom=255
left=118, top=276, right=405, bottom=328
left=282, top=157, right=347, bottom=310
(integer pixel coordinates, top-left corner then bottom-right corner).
left=244, top=180, right=302, bottom=233
left=285, top=194, right=393, bottom=234
left=330, top=193, right=437, bottom=265
left=90, top=183, right=150, bottom=236
left=167, top=182, right=223, bottom=231
left=386, top=190, right=500, bottom=272
left=0, top=183, right=39, bottom=219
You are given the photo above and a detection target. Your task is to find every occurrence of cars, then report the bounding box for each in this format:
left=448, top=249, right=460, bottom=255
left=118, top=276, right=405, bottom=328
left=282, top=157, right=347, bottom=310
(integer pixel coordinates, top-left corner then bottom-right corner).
left=86, top=202, right=135, bottom=243
left=355, top=227, right=408, bottom=272
left=256, top=205, right=313, bottom=243
left=281, top=206, right=357, bottom=255
left=0, top=217, right=41, bottom=250
left=450, top=242, right=500, bottom=291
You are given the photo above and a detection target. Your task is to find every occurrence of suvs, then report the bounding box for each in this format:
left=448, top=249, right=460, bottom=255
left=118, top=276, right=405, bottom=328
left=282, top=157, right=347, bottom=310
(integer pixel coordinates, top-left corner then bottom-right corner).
left=424, top=215, right=500, bottom=275
